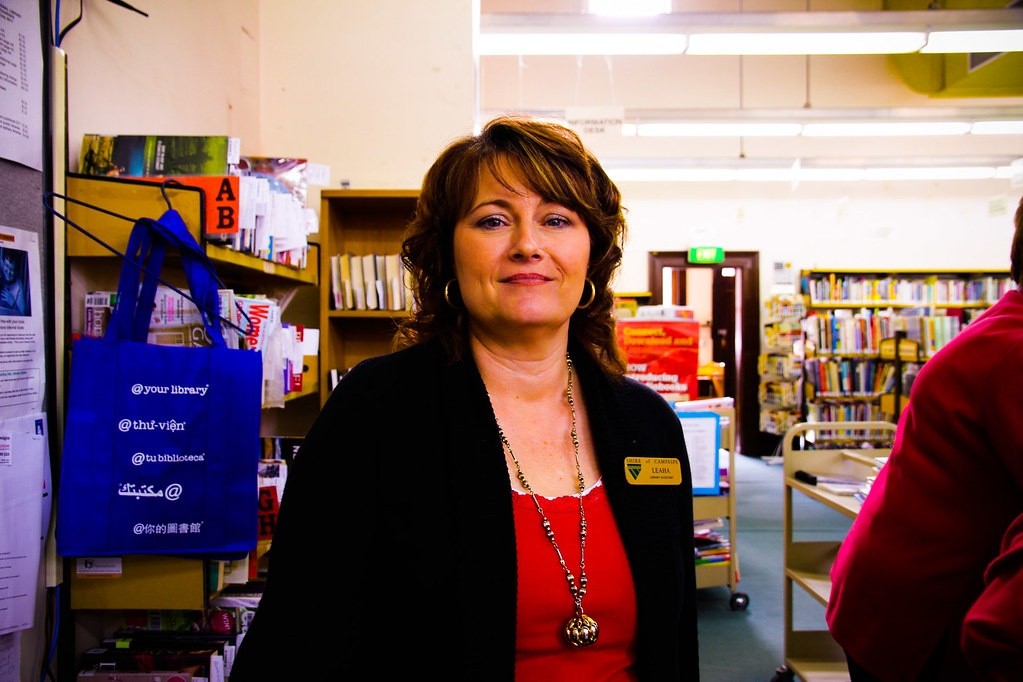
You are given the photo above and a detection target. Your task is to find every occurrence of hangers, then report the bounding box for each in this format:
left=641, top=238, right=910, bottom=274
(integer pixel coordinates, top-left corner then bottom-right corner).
left=44, top=179, right=252, bottom=334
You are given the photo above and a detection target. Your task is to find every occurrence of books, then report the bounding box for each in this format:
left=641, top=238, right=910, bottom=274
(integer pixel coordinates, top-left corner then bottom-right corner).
left=87, top=286, right=308, bottom=405
left=331, top=366, right=352, bottom=395
left=77, top=114, right=309, bottom=270
left=818, top=307, right=989, bottom=441
left=808, top=276, right=1018, bottom=303
left=330, top=253, right=420, bottom=312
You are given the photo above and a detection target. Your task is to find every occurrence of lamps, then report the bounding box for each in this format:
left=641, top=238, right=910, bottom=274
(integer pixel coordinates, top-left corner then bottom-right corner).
left=480, top=9, right=1023, bottom=186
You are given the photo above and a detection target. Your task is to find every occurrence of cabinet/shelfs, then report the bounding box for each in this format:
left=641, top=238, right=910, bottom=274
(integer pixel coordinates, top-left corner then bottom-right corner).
left=54, top=188, right=423, bottom=681
left=798, top=270, right=1015, bottom=450
left=771, top=422, right=900, bottom=681
left=669, top=400, right=750, bottom=611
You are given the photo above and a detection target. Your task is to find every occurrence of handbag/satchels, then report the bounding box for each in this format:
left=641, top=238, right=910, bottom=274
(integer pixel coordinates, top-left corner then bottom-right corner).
left=56, top=209, right=263, bottom=561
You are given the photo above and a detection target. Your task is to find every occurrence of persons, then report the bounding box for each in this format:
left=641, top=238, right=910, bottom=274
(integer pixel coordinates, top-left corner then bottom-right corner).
left=224, top=117, right=700, bottom=681
left=826, top=200, right=1023, bottom=682
left=0, top=256, right=25, bottom=314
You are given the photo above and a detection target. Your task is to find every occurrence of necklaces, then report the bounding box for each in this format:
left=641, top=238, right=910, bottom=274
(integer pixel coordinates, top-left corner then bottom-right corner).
left=486, top=351, right=599, bottom=650
